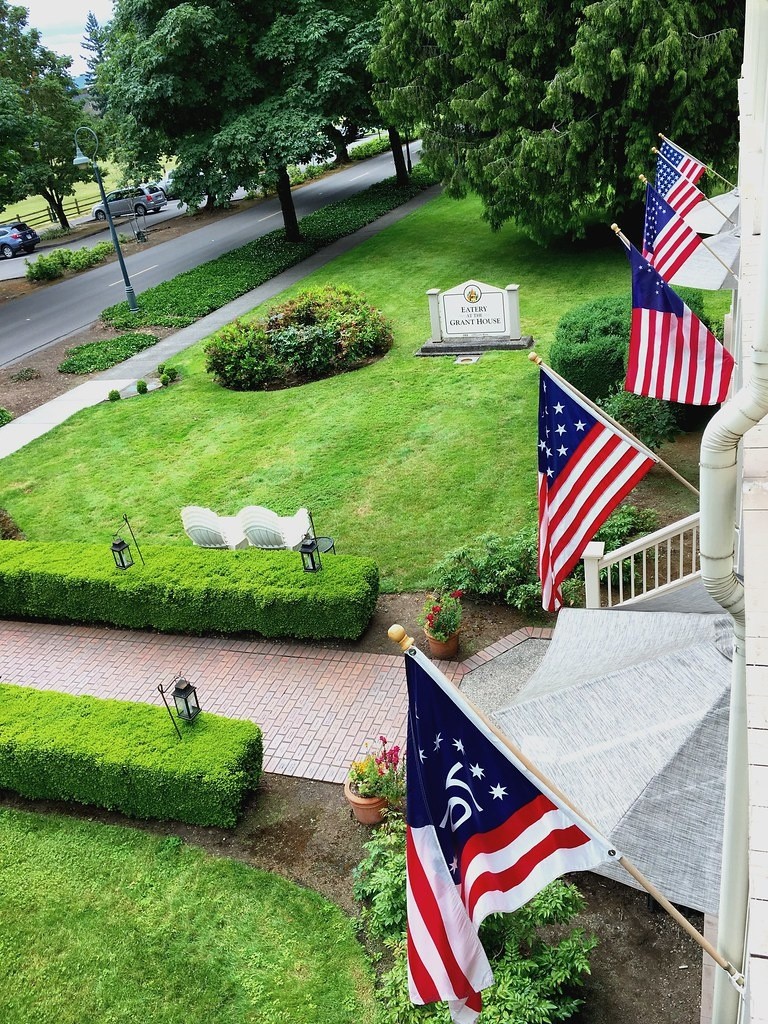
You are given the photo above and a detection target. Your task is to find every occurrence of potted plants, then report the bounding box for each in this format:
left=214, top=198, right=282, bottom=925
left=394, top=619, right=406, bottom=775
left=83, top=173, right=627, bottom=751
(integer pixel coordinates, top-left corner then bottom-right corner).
left=344, top=778, right=387, bottom=824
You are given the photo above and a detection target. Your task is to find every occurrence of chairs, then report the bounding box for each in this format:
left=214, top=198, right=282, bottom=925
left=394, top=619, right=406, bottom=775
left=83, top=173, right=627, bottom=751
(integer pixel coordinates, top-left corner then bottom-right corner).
left=236, top=505, right=314, bottom=552
left=180, top=506, right=248, bottom=550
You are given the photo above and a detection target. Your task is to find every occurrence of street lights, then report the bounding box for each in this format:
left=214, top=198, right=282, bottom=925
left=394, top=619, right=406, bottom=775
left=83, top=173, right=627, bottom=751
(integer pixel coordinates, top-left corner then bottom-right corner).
left=72, top=127, right=140, bottom=313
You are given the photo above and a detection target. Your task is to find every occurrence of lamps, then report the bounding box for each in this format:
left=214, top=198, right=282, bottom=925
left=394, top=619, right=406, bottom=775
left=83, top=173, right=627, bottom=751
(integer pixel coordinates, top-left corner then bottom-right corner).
left=171, top=676, right=201, bottom=726
left=110, top=533, right=135, bottom=570
left=297, top=540, right=323, bottom=575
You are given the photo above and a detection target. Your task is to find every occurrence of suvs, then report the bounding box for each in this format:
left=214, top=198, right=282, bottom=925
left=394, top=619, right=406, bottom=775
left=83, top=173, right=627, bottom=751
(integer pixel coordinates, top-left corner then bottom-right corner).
left=91, top=184, right=168, bottom=221
left=0, top=223, right=40, bottom=259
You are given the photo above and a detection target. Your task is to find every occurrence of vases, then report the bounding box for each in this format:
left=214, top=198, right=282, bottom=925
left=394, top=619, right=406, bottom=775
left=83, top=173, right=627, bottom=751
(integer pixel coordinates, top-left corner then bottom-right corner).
left=424, top=620, right=460, bottom=659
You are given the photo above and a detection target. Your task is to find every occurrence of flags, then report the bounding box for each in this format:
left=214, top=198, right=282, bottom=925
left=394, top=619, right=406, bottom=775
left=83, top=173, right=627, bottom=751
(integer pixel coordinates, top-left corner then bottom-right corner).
left=641, top=138, right=707, bottom=284
left=404, top=646, right=623, bottom=1024
left=616, top=233, right=734, bottom=406
left=537, top=366, right=660, bottom=613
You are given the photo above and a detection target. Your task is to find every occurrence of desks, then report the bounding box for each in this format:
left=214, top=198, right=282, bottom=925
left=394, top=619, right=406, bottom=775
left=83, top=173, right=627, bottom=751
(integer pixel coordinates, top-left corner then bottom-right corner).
left=308, top=536, right=336, bottom=554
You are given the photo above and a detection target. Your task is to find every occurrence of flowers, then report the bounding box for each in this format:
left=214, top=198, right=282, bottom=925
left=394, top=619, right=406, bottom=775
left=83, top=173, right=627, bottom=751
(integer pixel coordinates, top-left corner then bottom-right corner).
left=343, top=736, right=400, bottom=797
left=417, top=590, right=463, bottom=643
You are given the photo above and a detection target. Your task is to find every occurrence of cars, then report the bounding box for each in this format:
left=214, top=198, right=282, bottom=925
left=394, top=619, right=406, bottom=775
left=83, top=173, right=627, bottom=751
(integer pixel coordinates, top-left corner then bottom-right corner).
left=157, top=170, right=207, bottom=201
